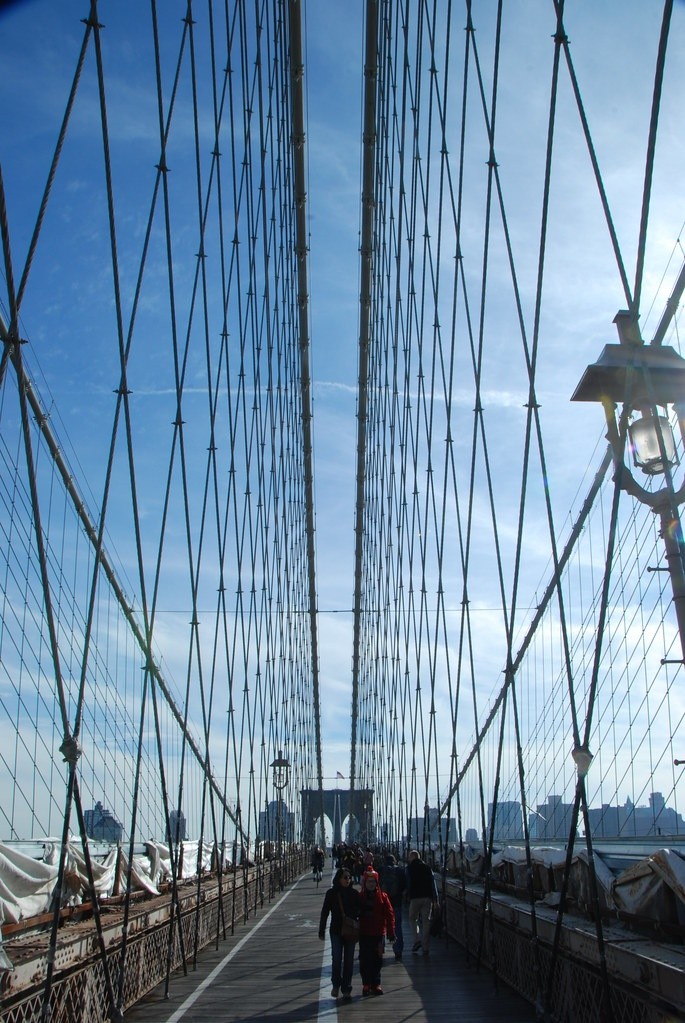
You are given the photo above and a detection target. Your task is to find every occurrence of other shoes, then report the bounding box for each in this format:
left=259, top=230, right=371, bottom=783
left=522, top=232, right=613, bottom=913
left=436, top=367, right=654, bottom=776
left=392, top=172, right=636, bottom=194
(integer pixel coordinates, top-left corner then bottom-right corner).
left=363, top=984, right=372, bottom=996
left=395, top=952, right=402, bottom=959
left=343, top=993, right=352, bottom=1001
left=331, top=986, right=339, bottom=997
left=371, top=984, right=383, bottom=996
left=412, top=941, right=422, bottom=951
left=423, top=949, right=429, bottom=954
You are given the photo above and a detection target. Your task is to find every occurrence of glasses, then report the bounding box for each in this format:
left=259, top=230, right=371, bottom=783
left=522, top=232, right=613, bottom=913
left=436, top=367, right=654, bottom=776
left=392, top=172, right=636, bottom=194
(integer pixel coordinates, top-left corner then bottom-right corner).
left=341, top=876, right=352, bottom=880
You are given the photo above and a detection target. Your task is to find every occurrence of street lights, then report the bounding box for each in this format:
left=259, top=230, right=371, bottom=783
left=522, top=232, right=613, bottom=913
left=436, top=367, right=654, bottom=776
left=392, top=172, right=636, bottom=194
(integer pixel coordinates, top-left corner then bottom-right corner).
left=270, top=750, right=292, bottom=859
left=569, top=305, right=684, bottom=664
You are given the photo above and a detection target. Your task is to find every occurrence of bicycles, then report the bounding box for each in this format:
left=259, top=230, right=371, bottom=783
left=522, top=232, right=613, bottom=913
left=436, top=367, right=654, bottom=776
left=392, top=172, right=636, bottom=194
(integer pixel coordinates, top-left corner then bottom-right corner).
left=311, top=864, right=323, bottom=889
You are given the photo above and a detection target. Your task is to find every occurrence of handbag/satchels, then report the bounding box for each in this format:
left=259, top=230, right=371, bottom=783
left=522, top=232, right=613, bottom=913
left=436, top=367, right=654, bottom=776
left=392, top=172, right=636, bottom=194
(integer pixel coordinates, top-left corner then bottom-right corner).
left=429, top=908, right=444, bottom=939
left=341, top=917, right=361, bottom=942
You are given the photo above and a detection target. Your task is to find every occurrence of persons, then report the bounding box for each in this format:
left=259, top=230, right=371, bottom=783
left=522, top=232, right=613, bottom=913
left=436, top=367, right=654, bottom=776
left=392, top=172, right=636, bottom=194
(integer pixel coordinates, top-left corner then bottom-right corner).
left=332, top=842, right=374, bottom=884
left=319, top=866, right=397, bottom=1001
left=378, top=850, right=440, bottom=960
left=311, top=844, right=325, bottom=881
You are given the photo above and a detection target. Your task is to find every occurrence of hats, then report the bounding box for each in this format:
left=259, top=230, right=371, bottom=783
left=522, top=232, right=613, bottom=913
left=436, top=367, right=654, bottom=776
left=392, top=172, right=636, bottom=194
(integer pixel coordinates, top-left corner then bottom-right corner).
left=362, top=866, right=379, bottom=885
